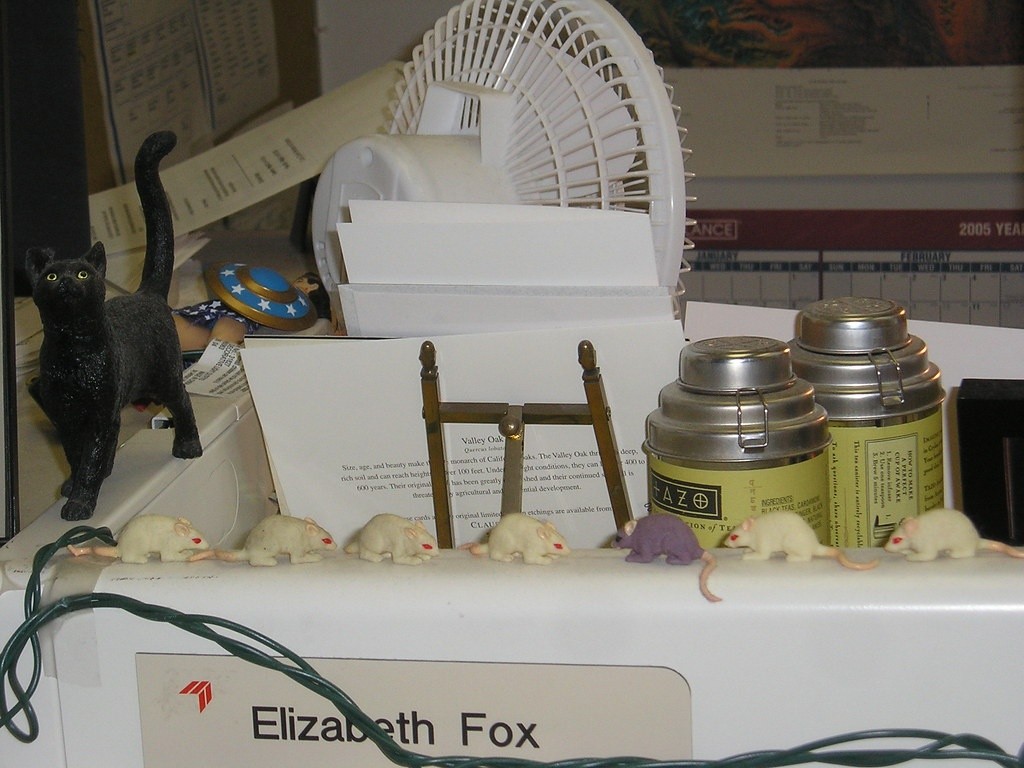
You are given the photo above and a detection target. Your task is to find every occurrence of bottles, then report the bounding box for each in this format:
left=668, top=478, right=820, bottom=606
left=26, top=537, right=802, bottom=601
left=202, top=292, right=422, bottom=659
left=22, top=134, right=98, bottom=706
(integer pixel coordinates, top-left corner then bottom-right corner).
left=790, top=293, right=946, bottom=550
left=641, top=334, right=830, bottom=547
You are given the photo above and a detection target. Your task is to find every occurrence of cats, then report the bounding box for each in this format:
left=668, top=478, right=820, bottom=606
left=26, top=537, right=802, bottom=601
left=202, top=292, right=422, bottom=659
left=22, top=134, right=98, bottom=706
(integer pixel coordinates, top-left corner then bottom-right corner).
left=25, top=130, right=204, bottom=521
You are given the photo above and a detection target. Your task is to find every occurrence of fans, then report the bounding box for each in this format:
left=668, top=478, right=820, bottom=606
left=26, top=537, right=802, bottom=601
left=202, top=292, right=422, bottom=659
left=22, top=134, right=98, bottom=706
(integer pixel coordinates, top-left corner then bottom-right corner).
left=313, top=0, right=697, bottom=320
